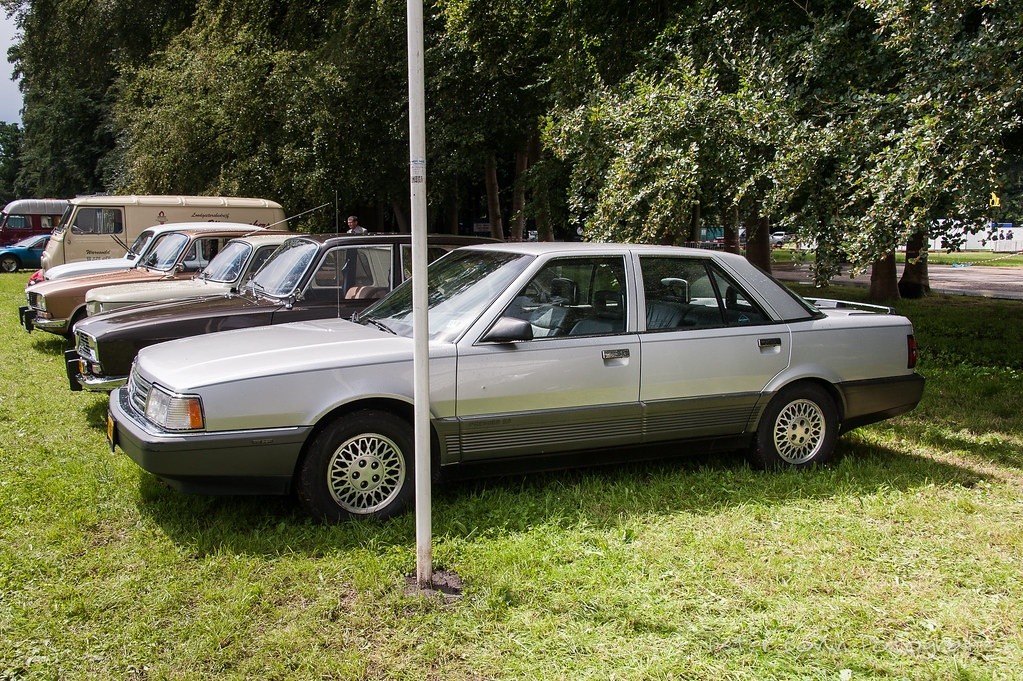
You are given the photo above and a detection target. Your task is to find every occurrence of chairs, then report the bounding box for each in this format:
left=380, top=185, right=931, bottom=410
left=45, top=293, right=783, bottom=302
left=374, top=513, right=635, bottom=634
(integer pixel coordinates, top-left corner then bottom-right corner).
left=646, top=278, right=689, bottom=331
left=528, top=278, right=576, bottom=340
left=691, top=285, right=766, bottom=327
left=569, top=291, right=626, bottom=336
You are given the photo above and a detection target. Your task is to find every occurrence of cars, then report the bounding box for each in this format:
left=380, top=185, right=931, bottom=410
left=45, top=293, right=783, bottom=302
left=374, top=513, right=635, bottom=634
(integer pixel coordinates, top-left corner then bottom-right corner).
left=86, top=234, right=315, bottom=316
left=42, top=220, right=267, bottom=286
left=24, top=227, right=256, bottom=348
left=769, top=232, right=792, bottom=245
left=0, top=234, right=52, bottom=272
left=103, top=240, right=926, bottom=536
left=64, top=233, right=490, bottom=398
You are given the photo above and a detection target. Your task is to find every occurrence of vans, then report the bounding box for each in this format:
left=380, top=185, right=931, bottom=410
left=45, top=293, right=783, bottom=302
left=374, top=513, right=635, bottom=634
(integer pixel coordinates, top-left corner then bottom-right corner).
left=38, top=192, right=289, bottom=273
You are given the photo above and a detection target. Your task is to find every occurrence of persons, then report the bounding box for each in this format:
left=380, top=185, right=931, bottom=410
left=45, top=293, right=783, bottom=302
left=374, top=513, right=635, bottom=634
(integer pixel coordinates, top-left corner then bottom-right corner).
left=346, top=216, right=367, bottom=235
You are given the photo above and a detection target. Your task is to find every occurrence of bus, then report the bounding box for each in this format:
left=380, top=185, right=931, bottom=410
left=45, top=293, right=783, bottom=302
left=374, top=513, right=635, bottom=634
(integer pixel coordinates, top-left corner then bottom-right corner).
left=0, top=199, right=69, bottom=245
